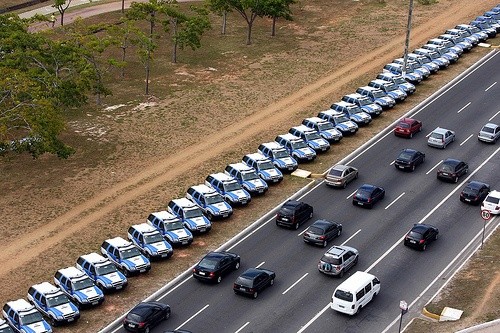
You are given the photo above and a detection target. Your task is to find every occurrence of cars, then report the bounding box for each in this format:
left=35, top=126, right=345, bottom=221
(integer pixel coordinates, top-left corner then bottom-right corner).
left=404, top=222, right=440, bottom=250
left=437, top=158, right=469, bottom=184
left=477, top=122, right=500, bottom=143
left=394, top=147, right=426, bottom=172
left=122, top=302, right=171, bottom=333
left=481, top=190, right=500, bottom=213
left=427, top=128, right=459, bottom=149
left=233, top=267, right=277, bottom=297
left=192, top=251, right=244, bottom=284
left=304, top=219, right=343, bottom=249
left=325, top=165, right=360, bottom=189
left=393, top=117, right=423, bottom=138
left=353, top=184, right=386, bottom=210
left=460, top=181, right=493, bottom=204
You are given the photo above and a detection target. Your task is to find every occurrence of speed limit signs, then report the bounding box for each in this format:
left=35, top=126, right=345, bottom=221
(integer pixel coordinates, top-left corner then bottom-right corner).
left=481, top=210, right=491, bottom=220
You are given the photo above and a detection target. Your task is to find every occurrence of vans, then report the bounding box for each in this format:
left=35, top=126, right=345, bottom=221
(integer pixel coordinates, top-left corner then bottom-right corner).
left=329, top=271, right=381, bottom=316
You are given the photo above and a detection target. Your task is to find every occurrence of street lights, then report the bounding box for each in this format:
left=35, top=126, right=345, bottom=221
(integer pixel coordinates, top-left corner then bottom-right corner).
left=398, top=300, right=409, bottom=333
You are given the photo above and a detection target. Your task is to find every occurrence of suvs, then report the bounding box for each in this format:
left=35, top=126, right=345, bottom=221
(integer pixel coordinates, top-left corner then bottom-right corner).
left=1, top=4, right=500, bottom=333
left=276, top=199, right=316, bottom=231
left=317, top=245, right=359, bottom=278
